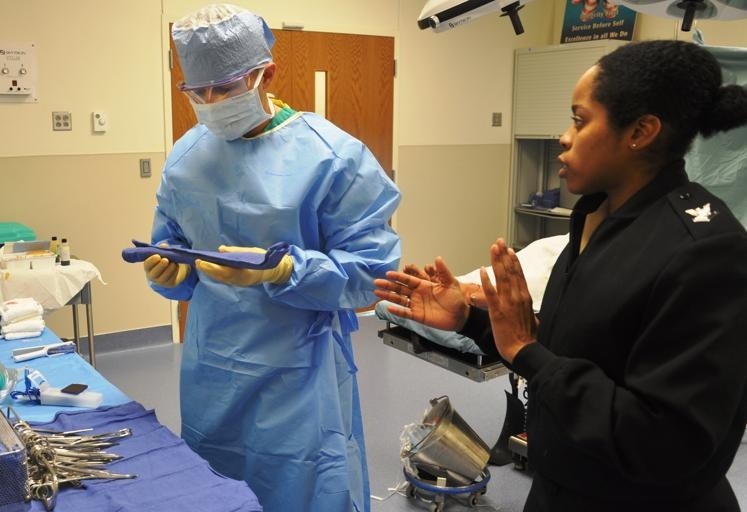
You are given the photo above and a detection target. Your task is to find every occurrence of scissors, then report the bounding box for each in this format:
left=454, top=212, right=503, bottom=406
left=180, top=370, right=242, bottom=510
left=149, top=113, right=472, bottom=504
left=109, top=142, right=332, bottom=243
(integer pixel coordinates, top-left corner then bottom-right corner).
left=13, top=422, right=138, bottom=501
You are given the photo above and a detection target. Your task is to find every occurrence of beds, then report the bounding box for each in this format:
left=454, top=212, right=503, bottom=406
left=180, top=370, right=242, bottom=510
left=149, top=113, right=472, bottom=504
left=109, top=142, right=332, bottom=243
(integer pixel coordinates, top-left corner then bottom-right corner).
left=374, top=234, right=576, bottom=382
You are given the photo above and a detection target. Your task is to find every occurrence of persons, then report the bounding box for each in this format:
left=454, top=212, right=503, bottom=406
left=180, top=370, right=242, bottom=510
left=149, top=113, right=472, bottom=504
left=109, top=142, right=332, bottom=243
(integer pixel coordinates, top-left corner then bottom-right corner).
left=373, top=39, right=746, bottom=512
left=396, top=261, right=541, bottom=331
left=143, top=4, right=403, bottom=512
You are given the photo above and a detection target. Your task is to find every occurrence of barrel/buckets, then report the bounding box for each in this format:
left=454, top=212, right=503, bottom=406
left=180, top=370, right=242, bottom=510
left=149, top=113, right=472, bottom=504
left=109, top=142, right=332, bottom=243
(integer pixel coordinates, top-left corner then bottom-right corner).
left=402, top=394, right=492, bottom=486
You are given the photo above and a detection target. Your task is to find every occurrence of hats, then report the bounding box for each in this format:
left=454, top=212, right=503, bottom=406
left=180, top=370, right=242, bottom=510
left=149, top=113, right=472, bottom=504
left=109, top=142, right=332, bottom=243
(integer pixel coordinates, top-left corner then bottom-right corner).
left=171, top=4, right=276, bottom=85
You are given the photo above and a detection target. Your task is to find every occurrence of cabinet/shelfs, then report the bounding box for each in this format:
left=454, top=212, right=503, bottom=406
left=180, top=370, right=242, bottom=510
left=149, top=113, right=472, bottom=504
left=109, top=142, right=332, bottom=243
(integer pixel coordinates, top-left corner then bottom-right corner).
left=505, top=40, right=747, bottom=254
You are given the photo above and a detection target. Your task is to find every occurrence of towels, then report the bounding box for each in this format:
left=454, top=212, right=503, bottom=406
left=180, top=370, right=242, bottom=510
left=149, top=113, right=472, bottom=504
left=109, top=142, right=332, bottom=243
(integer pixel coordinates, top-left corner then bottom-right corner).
left=0, top=297, right=45, bottom=340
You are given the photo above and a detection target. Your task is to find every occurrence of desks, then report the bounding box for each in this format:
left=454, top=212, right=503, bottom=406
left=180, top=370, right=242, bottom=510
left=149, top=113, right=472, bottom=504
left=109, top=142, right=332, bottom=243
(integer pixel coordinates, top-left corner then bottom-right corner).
left=0, top=323, right=260, bottom=511
left=0, top=253, right=95, bottom=371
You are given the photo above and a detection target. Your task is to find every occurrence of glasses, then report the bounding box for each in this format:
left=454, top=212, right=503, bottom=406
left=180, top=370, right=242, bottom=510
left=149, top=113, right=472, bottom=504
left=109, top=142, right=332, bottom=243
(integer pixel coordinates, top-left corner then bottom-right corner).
left=177, top=64, right=264, bottom=104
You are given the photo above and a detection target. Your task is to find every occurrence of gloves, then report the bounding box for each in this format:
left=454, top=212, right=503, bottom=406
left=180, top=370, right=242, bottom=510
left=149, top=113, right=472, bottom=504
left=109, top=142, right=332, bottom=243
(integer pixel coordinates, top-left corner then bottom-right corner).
left=143, top=243, right=191, bottom=287
left=195, top=244, right=293, bottom=287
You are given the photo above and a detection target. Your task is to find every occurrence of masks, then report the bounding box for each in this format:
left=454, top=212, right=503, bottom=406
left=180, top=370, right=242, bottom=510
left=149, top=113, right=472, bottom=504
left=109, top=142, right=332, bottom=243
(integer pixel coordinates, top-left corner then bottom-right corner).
left=189, top=89, right=274, bottom=141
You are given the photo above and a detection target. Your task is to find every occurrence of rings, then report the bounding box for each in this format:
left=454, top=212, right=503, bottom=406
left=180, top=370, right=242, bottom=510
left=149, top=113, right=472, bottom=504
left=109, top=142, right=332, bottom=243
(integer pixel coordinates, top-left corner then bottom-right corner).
left=405, top=297, right=410, bottom=308
